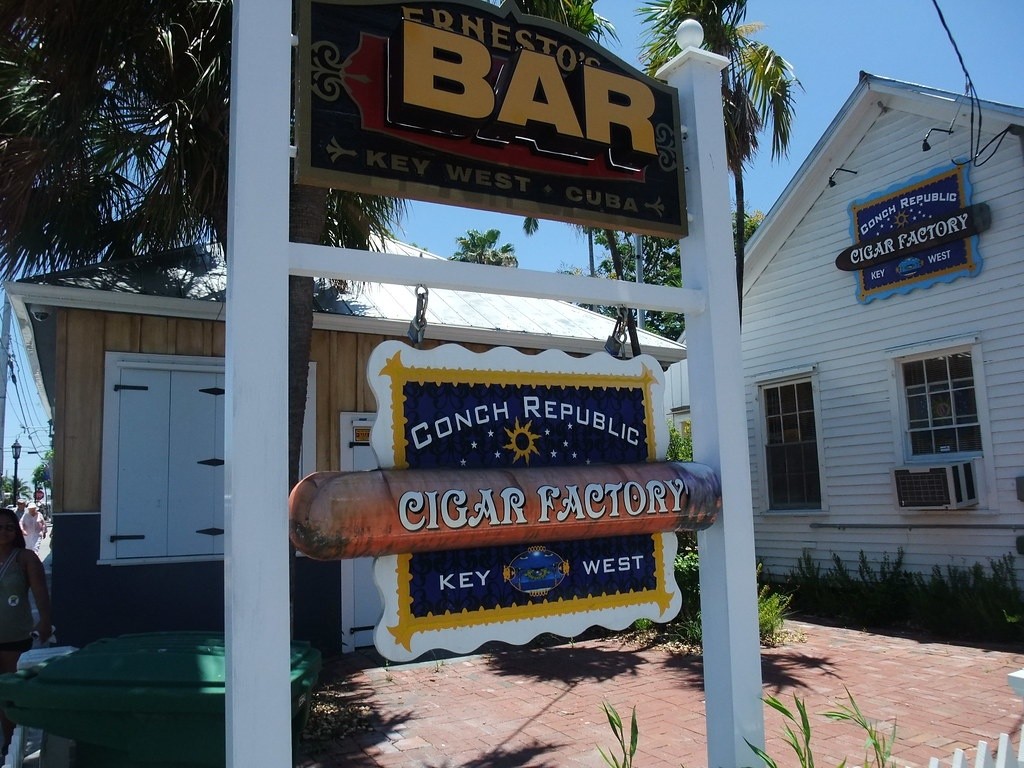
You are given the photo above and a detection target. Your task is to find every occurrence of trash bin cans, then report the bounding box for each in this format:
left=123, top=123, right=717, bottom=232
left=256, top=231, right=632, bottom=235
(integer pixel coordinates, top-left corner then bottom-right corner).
left=0, top=628, right=323, bottom=768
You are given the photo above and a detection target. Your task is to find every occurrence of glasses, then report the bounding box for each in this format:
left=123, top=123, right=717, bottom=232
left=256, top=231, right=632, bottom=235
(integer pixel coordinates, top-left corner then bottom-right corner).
left=0, top=526, right=17, bottom=532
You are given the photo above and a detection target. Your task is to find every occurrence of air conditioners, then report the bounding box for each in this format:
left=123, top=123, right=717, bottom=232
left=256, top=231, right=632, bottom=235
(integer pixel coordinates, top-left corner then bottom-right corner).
left=889, top=461, right=980, bottom=512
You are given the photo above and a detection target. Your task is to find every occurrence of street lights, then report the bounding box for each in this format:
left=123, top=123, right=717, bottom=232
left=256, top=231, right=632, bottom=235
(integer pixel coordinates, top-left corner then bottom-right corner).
left=11, top=439, right=22, bottom=507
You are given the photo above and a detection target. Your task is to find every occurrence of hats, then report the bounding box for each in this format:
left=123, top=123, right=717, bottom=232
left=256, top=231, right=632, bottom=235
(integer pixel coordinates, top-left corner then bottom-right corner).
left=26, top=503, right=40, bottom=511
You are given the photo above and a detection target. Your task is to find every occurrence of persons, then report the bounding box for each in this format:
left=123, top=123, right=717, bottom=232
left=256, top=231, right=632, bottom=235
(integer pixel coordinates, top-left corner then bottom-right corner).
left=14, top=499, right=27, bottom=522
left=19, top=502, right=48, bottom=557
left=0, top=509, right=53, bottom=768
